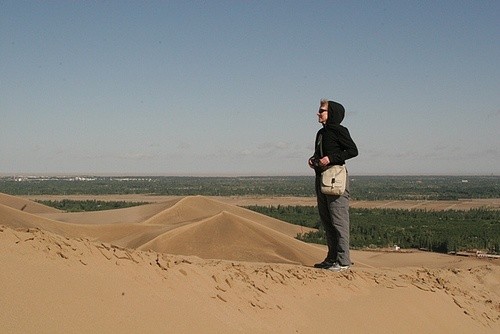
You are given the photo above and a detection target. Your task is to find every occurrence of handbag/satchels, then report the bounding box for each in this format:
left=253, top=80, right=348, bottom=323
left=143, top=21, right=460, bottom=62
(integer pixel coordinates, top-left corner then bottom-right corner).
left=320, top=164, right=346, bottom=195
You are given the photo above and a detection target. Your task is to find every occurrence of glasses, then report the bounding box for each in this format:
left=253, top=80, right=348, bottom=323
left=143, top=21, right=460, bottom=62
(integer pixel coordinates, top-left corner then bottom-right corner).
left=319, top=109, right=327, bottom=113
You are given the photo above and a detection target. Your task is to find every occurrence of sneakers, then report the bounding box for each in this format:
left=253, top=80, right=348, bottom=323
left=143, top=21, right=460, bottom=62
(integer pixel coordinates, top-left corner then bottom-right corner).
left=313, top=258, right=333, bottom=268
left=328, top=262, right=351, bottom=272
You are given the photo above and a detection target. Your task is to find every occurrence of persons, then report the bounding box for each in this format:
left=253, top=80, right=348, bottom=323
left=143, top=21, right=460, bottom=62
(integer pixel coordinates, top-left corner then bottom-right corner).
left=308, top=98, right=359, bottom=272
